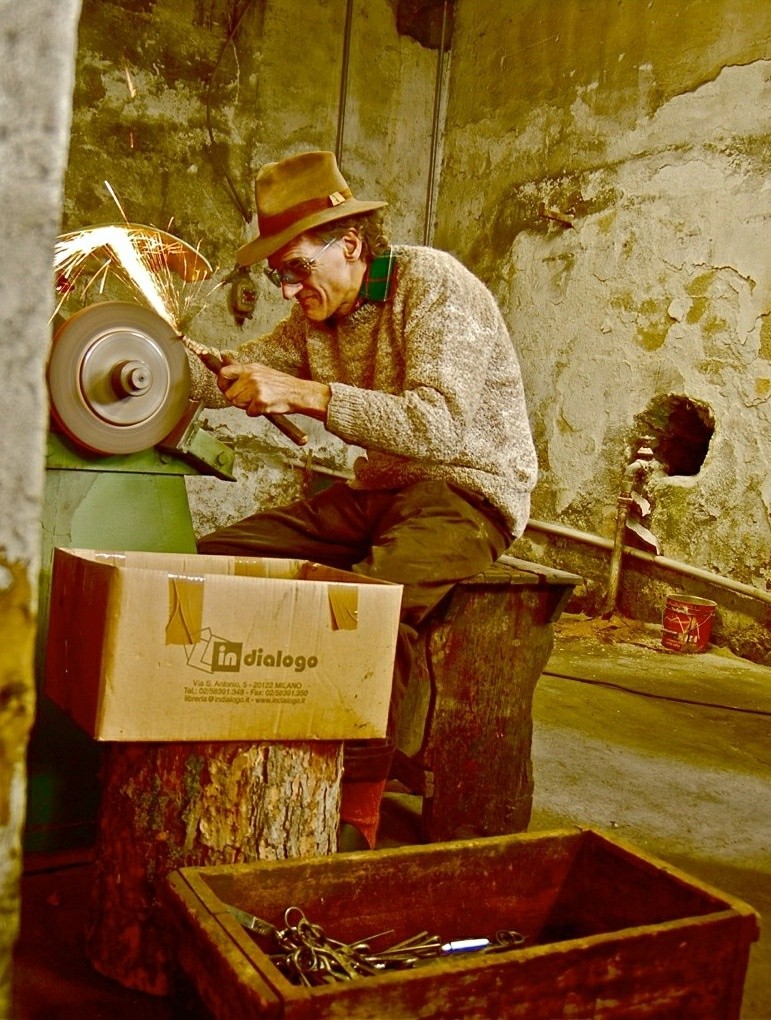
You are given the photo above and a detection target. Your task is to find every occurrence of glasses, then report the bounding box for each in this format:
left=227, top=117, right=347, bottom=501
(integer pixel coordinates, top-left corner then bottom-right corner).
left=264, top=237, right=338, bottom=288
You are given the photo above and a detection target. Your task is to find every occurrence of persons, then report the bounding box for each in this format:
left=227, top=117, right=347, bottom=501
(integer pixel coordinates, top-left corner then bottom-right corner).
left=186, top=152, right=540, bottom=854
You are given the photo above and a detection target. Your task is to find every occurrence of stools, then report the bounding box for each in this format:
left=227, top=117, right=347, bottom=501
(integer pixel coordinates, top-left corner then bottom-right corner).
left=389, top=556, right=588, bottom=843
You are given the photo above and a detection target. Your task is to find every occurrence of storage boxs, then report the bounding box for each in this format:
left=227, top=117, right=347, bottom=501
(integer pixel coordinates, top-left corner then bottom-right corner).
left=44, top=546, right=403, bottom=743
left=158, top=821, right=766, bottom=1017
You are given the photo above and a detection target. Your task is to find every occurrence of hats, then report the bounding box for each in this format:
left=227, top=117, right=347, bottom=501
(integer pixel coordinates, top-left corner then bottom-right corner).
left=234, top=151, right=390, bottom=268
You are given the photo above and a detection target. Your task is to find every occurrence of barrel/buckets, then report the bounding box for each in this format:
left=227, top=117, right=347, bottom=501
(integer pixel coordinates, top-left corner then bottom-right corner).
left=661, top=592, right=716, bottom=652
